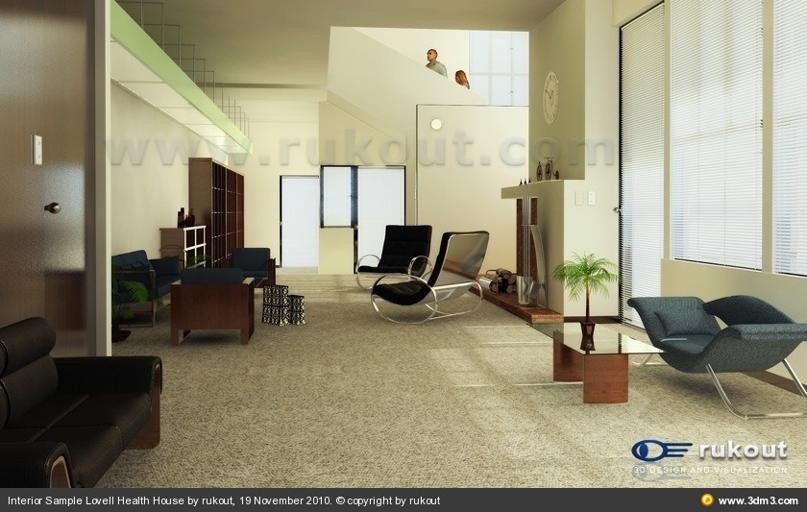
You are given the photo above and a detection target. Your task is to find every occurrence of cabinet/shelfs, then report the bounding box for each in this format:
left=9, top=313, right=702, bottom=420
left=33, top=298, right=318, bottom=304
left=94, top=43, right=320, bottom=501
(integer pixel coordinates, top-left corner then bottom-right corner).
left=226, top=167, right=238, bottom=260
left=189, top=157, right=226, bottom=268
left=160, top=226, right=207, bottom=269
left=238, top=174, right=244, bottom=248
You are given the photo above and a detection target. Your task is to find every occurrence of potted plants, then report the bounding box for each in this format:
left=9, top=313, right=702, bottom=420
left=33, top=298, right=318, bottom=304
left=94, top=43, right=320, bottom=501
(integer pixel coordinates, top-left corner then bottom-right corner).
left=552, top=249, right=620, bottom=336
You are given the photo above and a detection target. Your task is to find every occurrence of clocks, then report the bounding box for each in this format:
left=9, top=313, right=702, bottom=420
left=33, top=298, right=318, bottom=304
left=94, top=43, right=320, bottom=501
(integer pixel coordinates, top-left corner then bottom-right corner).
left=543, top=72, right=560, bottom=124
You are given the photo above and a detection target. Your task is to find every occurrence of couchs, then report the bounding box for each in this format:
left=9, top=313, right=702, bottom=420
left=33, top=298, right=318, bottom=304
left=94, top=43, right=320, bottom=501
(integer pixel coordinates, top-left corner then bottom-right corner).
left=0, top=317, right=163, bottom=487
left=112, top=250, right=183, bottom=327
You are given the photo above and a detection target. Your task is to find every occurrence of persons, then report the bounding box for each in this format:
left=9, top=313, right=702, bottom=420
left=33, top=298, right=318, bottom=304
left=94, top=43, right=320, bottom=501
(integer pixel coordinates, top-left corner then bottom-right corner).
left=455, top=69, right=470, bottom=90
left=424, top=48, right=447, bottom=79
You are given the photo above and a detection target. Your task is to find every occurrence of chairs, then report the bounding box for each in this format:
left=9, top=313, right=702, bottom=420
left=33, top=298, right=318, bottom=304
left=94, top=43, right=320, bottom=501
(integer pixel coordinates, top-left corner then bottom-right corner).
left=355, top=226, right=434, bottom=292
left=628, top=295, right=807, bottom=420
left=170, top=269, right=255, bottom=345
left=224, top=248, right=276, bottom=288
left=370, top=231, right=490, bottom=324
